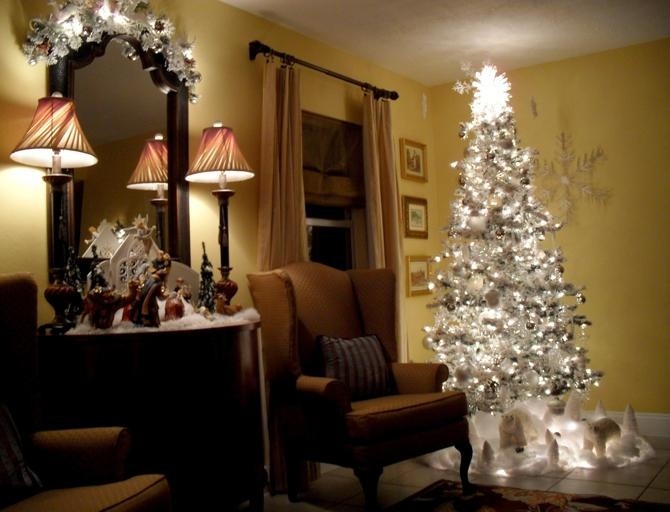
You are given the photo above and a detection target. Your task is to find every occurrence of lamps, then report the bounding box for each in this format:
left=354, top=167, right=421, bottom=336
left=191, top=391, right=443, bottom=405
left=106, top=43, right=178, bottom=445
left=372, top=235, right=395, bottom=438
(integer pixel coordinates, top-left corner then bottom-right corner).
left=125, top=132, right=168, bottom=255
left=185, top=120, right=256, bottom=306
left=9, top=90, right=100, bottom=333
left=423, top=59, right=605, bottom=413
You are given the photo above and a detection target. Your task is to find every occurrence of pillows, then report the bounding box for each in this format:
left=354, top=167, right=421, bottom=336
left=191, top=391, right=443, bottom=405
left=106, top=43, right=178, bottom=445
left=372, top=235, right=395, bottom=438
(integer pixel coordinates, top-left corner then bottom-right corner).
left=313, top=335, right=399, bottom=402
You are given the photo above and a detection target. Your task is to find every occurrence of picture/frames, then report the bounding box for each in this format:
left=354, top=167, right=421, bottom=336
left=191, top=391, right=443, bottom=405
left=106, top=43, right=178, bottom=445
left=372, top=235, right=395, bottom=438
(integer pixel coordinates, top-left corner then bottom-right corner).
left=398, top=136, right=434, bottom=295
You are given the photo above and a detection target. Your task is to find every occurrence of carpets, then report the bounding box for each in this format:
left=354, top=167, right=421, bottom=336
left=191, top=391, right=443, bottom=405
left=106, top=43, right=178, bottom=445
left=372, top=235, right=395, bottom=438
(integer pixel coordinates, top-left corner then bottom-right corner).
left=380, top=479, right=669, bottom=511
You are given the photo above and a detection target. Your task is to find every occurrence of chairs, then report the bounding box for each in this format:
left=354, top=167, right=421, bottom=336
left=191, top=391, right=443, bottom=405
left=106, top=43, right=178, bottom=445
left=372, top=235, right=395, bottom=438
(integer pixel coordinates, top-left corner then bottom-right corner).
left=0, top=271, right=171, bottom=511
left=245, top=261, right=474, bottom=511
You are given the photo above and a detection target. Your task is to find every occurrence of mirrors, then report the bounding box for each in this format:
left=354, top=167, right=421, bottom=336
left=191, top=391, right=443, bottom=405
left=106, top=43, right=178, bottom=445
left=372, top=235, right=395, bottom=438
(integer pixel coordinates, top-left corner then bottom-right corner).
left=21, top=0, right=202, bottom=288
